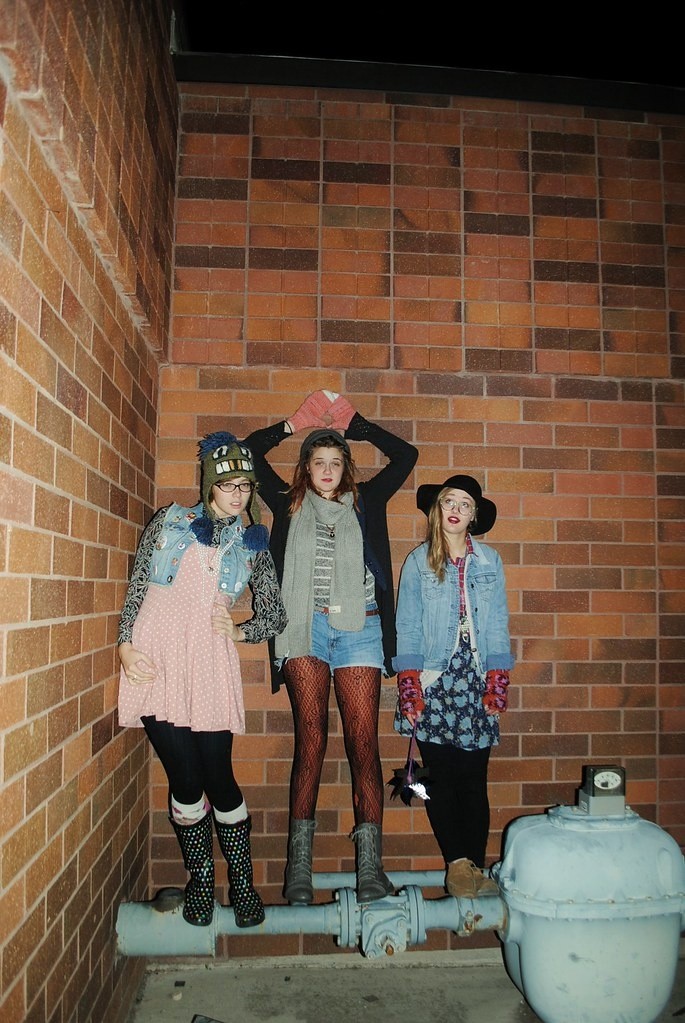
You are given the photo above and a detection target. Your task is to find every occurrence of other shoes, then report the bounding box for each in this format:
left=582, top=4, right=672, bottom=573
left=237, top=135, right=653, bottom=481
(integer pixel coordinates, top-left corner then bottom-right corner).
left=471, top=867, right=500, bottom=895
left=446, top=858, right=477, bottom=899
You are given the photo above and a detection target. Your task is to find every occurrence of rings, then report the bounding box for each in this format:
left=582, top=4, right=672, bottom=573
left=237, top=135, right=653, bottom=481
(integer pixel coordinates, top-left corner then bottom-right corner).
left=132, top=676, right=137, bottom=681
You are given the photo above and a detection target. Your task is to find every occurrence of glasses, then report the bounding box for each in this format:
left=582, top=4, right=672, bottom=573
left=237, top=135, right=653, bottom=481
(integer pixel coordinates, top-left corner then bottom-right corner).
left=441, top=498, right=475, bottom=516
left=214, top=482, right=255, bottom=492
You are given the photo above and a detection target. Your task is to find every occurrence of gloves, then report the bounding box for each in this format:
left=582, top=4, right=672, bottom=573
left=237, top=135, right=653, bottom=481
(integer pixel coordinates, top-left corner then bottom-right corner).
left=288, top=390, right=335, bottom=434
left=328, top=392, right=356, bottom=430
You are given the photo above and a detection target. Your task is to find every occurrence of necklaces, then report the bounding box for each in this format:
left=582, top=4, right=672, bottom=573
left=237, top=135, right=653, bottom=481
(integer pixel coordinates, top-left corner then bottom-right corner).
left=194, top=534, right=235, bottom=606
left=326, top=524, right=336, bottom=537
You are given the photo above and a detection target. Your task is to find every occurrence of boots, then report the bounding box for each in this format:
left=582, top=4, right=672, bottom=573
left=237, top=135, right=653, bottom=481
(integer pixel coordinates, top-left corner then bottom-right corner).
left=168, top=811, right=214, bottom=926
left=213, top=816, right=265, bottom=928
left=283, top=816, right=319, bottom=904
left=350, top=823, right=394, bottom=903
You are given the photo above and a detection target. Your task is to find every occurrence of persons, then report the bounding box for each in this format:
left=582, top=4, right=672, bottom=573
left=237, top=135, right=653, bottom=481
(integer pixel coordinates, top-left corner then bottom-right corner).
left=117, top=430, right=289, bottom=928
left=239, top=389, right=418, bottom=905
left=391, top=475, right=515, bottom=896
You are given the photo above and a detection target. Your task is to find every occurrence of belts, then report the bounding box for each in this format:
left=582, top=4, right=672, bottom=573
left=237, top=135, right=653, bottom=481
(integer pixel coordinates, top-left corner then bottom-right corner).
left=314, top=606, right=380, bottom=616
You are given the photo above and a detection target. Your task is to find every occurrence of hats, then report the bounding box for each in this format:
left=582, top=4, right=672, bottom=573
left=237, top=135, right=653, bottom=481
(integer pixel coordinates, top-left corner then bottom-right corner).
left=300, top=428, right=352, bottom=473
left=416, top=475, right=497, bottom=536
left=189, top=431, right=270, bottom=552
